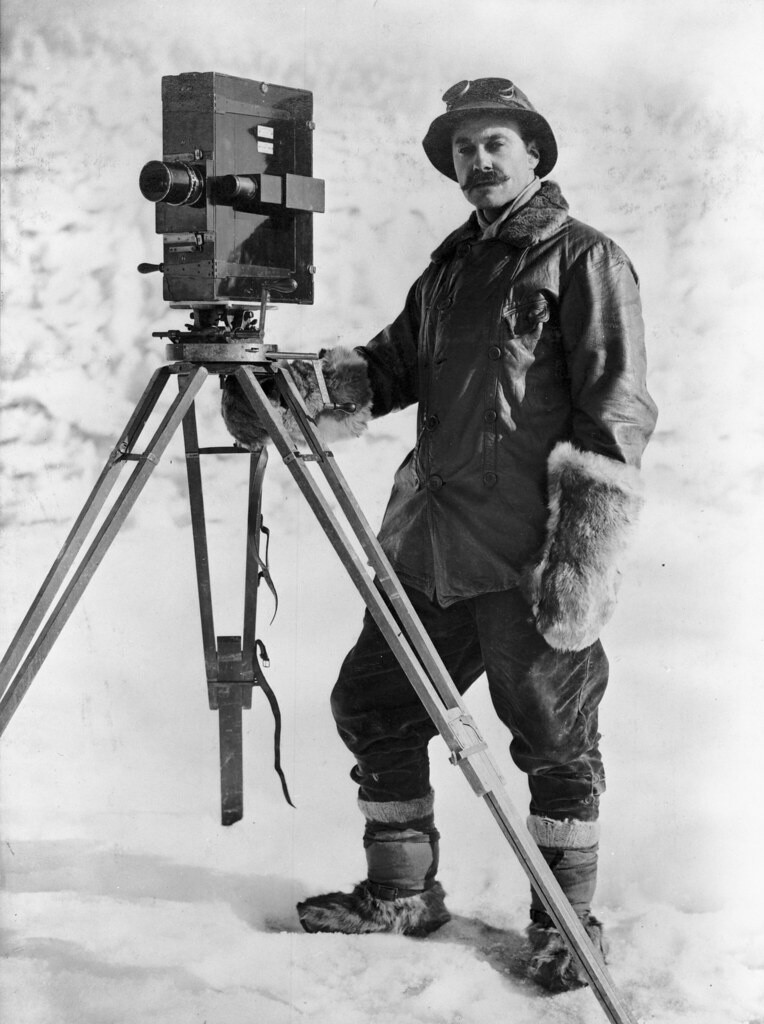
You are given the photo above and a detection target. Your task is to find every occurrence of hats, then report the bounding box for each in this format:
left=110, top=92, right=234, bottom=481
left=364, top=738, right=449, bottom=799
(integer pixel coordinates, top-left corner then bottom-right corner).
left=423, top=78, right=558, bottom=183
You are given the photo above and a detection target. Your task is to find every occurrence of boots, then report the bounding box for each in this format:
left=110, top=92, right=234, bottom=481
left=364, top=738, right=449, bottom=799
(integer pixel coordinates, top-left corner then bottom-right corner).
left=510, top=815, right=609, bottom=993
left=296, top=785, right=451, bottom=938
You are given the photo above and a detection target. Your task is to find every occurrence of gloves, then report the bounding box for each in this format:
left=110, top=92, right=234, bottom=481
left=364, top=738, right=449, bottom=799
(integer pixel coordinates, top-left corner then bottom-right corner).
left=533, top=440, right=649, bottom=652
left=220, top=345, right=374, bottom=451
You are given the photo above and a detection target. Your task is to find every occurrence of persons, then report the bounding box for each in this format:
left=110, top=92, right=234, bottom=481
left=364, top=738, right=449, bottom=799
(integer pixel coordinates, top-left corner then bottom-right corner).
left=223, top=77, right=659, bottom=994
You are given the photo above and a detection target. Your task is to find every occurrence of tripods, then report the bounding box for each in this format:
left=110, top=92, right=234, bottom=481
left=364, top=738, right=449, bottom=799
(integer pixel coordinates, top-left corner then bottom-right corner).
left=0, top=302, right=642, bottom=1024
